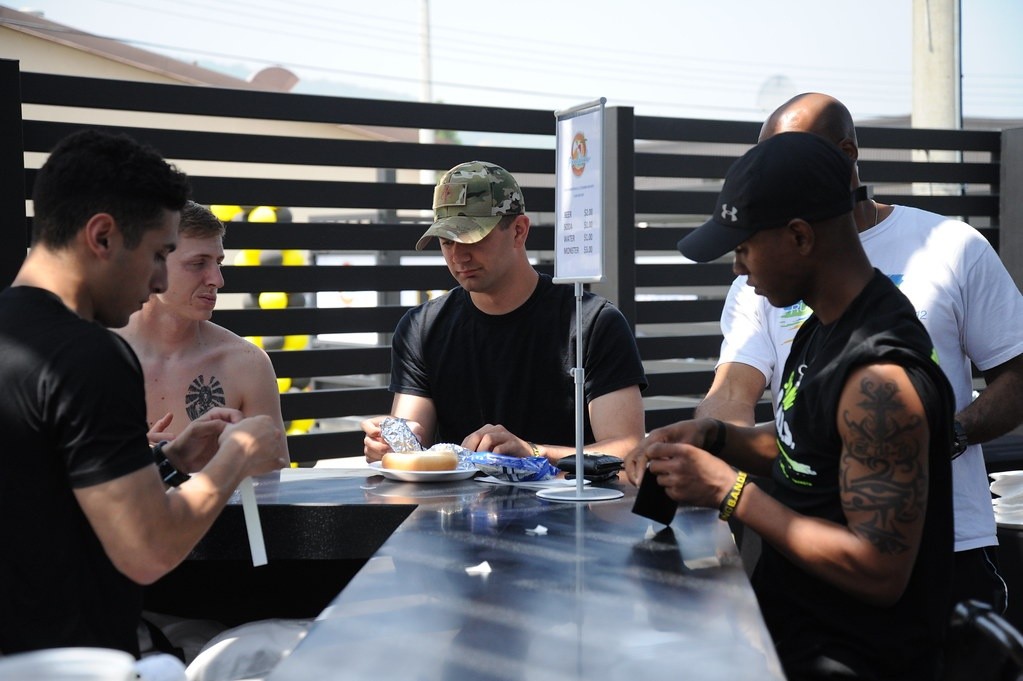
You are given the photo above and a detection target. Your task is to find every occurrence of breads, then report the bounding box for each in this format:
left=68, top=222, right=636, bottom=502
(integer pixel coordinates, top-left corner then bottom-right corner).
left=382, top=450, right=458, bottom=471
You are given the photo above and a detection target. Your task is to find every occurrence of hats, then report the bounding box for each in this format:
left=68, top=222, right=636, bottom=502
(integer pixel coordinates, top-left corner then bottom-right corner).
left=677, top=131, right=869, bottom=263
left=416, top=161, right=525, bottom=251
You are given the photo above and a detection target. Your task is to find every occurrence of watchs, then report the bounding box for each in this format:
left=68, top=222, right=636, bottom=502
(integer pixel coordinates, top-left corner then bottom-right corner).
left=152, top=440, right=191, bottom=488
left=951, top=419, right=968, bottom=460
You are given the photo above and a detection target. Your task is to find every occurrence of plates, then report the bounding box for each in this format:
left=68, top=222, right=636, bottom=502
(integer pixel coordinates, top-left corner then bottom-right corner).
left=368, top=461, right=478, bottom=483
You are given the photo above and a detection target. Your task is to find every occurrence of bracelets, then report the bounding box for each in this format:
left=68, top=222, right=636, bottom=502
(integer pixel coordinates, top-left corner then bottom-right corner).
left=709, top=419, right=726, bottom=454
left=527, top=442, right=540, bottom=456
left=718, top=471, right=752, bottom=521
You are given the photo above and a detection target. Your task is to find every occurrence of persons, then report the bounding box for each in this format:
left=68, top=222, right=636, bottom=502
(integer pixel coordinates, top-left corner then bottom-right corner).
left=628, top=132, right=956, bottom=681
left=108, top=199, right=291, bottom=470
left=695, top=92, right=1023, bottom=620
left=360, top=161, right=647, bottom=492
left=0, top=133, right=291, bottom=661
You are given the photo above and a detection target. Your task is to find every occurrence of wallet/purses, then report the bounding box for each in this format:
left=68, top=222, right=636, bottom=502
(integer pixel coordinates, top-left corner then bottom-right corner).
left=557, top=453, right=626, bottom=474
